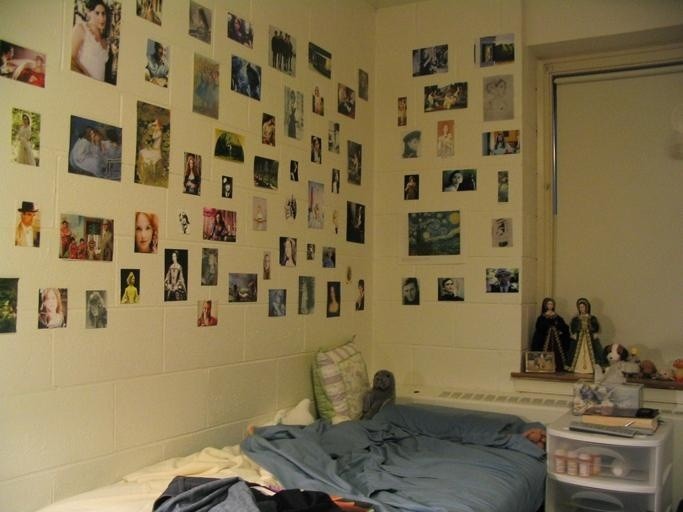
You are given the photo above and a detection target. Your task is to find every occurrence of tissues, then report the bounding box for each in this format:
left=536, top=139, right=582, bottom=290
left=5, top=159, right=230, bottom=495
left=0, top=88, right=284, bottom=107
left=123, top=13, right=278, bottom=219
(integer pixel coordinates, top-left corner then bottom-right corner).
left=572, top=364, right=644, bottom=409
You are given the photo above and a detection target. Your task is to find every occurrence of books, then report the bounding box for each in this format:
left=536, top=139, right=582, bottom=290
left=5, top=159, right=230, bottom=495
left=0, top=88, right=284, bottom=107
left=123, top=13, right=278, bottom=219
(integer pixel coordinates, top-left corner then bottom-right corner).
left=581, top=406, right=661, bottom=431
left=566, top=414, right=660, bottom=436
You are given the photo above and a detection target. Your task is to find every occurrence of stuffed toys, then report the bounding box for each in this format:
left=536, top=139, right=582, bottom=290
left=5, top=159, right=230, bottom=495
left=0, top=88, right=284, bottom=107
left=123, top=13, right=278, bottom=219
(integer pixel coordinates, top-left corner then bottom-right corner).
left=602, top=341, right=683, bottom=384
left=361, top=370, right=396, bottom=419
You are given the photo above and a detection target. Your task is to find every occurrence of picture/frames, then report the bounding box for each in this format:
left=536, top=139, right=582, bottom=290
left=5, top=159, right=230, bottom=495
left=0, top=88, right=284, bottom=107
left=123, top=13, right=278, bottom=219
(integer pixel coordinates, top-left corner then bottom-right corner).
left=525, top=351, right=556, bottom=374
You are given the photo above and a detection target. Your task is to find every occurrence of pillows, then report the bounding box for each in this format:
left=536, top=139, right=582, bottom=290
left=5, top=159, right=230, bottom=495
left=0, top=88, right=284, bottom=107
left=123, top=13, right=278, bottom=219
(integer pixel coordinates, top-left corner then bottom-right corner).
left=311, top=332, right=370, bottom=425
left=383, top=401, right=525, bottom=447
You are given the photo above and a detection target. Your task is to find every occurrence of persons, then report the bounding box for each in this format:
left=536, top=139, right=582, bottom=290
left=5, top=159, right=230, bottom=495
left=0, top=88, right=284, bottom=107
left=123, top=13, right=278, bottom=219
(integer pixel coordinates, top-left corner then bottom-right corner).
left=571, top=297, right=601, bottom=373
left=0, top=0, right=125, bottom=90
left=165, top=158, right=364, bottom=327
left=398, top=42, right=516, bottom=301
left=0, top=101, right=164, bottom=329
left=533, top=297, right=574, bottom=372
left=142, top=4, right=368, bottom=165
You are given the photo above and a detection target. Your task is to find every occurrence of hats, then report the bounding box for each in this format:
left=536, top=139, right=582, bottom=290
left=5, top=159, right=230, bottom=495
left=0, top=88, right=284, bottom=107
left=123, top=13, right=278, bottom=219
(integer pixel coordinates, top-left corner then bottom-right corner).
left=18, top=201, right=40, bottom=212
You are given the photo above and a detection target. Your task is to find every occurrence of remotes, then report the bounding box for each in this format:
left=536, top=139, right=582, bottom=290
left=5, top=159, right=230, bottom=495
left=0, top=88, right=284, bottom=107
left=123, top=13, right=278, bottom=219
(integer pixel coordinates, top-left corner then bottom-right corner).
left=569, top=421, right=636, bottom=437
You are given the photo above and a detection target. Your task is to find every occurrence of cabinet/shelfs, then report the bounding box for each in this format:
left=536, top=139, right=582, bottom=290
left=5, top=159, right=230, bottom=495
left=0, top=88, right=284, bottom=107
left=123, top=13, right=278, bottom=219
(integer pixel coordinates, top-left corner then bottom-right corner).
left=545, top=408, right=674, bottom=512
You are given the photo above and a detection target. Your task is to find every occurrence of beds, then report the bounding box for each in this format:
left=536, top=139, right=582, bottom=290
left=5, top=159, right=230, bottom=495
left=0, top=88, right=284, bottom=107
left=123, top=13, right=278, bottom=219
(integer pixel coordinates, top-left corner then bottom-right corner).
left=35, top=421, right=546, bottom=512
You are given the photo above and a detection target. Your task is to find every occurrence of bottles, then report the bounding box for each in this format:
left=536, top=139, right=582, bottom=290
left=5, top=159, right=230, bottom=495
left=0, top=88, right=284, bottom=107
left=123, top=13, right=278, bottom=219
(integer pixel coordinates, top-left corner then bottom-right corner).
left=630, top=347, right=642, bottom=368
left=611, top=453, right=636, bottom=478
left=552, top=449, right=602, bottom=479
